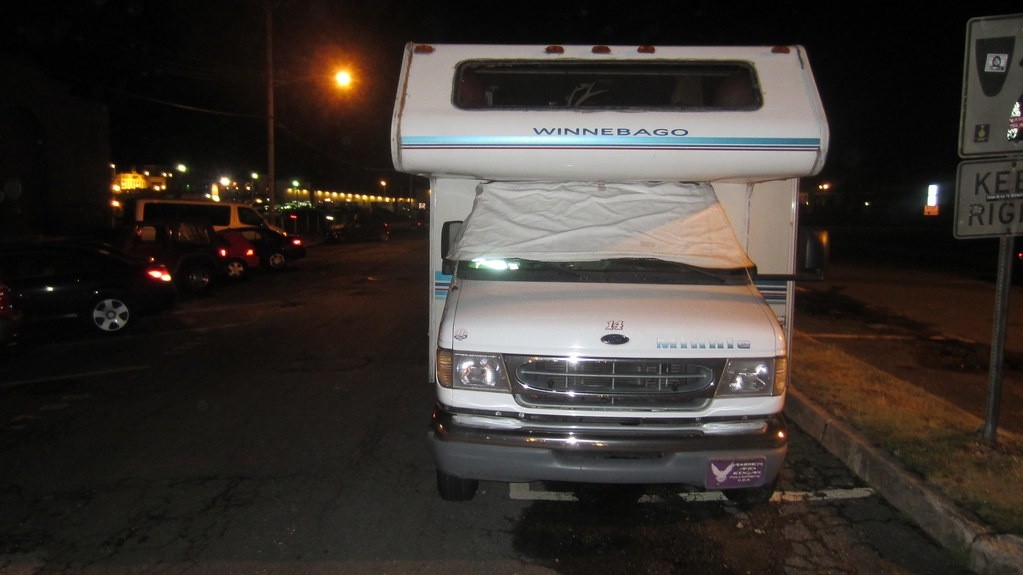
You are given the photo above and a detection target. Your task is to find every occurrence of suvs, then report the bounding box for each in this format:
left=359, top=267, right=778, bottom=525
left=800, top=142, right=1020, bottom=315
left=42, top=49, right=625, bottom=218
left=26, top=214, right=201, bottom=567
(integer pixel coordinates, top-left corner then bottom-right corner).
left=97, top=217, right=215, bottom=296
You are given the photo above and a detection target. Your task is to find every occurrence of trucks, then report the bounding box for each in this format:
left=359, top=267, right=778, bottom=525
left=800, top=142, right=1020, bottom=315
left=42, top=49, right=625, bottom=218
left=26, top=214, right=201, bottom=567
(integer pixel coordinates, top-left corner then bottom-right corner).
left=392, top=41, right=830, bottom=502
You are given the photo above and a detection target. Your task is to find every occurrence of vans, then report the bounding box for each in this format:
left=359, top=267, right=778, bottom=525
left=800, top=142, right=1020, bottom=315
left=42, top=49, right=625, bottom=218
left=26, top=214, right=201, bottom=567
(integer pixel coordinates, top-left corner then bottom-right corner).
left=133, top=199, right=287, bottom=245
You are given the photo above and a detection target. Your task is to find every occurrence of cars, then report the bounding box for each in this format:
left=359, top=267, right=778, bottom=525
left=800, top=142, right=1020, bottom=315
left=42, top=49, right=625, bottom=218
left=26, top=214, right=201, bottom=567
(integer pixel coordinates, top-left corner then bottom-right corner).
left=287, top=206, right=422, bottom=243
left=213, top=227, right=306, bottom=282
left=0, top=236, right=175, bottom=336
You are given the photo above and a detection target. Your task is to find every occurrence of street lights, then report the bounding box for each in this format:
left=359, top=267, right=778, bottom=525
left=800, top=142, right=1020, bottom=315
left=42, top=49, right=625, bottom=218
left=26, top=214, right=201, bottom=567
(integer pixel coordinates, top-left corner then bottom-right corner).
left=267, top=73, right=350, bottom=226
